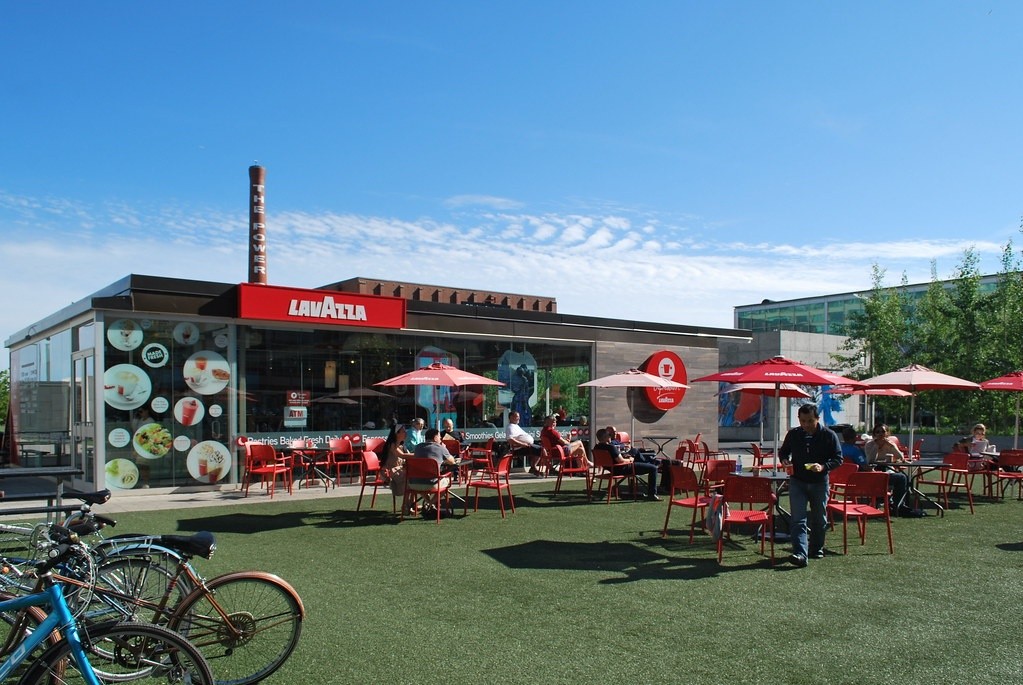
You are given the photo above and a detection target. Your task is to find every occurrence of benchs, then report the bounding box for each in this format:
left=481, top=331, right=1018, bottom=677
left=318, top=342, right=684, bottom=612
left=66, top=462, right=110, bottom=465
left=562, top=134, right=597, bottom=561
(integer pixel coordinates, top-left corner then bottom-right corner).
left=0, top=492, right=85, bottom=525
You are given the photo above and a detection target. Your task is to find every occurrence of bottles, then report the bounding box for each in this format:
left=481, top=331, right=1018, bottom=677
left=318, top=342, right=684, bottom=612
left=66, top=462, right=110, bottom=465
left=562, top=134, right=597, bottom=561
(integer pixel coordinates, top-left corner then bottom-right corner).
left=736, top=455, right=743, bottom=474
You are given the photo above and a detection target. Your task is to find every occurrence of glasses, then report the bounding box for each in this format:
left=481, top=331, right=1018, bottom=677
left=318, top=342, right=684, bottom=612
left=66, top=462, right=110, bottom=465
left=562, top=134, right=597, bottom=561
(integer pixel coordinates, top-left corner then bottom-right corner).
left=875, top=430, right=886, bottom=434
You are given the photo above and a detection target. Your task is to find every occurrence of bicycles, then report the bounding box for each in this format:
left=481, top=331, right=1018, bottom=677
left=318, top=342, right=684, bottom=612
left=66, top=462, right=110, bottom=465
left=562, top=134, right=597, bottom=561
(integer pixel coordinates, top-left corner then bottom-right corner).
left=0, top=488, right=304, bottom=685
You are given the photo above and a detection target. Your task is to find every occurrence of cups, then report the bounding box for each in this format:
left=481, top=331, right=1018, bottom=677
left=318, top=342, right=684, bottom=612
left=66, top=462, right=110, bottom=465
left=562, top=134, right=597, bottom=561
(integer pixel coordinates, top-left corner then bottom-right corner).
left=183, top=401, right=198, bottom=425
left=886, top=454, right=894, bottom=462
left=615, top=445, right=620, bottom=451
left=624, top=446, right=630, bottom=452
left=455, top=458, right=462, bottom=464
left=752, top=466, right=761, bottom=476
left=785, top=464, right=794, bottom=476
left=805, top=463, right=813, bottom=470
left=308, top=438, right=312, bottom=448
left=107, top=319, right=143, bottom=351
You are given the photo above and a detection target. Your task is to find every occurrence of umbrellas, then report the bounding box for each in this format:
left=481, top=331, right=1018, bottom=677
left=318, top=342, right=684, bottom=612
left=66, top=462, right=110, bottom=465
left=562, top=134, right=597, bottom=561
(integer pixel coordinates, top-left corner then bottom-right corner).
left=373, top=360, right=507, bottom=430
left=851, top=361, right=982, bottom=491
left=713, top=381, right=811, bottom=463
left=692, top=355, right=872, bottom=522
left=576, top=367, right=690, bottom=491
left=824, top=385, right=917, bottom=434
left=979, top=370, right=1023, bottom=499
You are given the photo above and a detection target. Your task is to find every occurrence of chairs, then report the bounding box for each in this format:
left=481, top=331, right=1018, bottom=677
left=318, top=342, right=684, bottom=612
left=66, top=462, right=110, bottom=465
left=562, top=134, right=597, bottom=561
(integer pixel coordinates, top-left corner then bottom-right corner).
left=238, top=433, right=1023, bottom=565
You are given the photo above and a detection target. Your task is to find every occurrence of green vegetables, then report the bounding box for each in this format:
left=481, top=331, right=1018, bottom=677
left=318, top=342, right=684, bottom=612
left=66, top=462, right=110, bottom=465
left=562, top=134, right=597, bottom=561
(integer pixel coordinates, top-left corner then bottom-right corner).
left=136, top=425, right=172, bottom=456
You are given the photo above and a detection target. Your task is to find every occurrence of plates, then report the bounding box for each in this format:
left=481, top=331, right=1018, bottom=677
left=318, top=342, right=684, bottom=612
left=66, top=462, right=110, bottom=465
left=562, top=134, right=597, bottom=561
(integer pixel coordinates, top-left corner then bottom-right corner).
left=173, top=321, right=200, bottom=346
left=105, top=458, right=139, bottom=489
left=174, top=396, right=205, bottom=425
left=104, top=364, right=152, bottom=410
left=133, top=422, right=173, bottom=459
left=187, top=440, right=232, bottom=484
left=183, top=350, right=230, bottom=395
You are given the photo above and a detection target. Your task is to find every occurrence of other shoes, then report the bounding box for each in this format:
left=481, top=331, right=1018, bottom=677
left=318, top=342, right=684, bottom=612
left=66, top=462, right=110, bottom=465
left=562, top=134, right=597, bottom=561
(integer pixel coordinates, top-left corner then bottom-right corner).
left=893, top=505, right=912, bottom=515
left=647, top=493, right=664, bottom=501
left=549, top=468, right=559, bottom=476
left=402, top=504, right=417, bottom=516
left=788, top=554, right=808, bottom=566
left=813, top=549, right=824, bottom=558
left=528, top=467, right=544, bottom=476
left=423, top=504, right=438, bottom=517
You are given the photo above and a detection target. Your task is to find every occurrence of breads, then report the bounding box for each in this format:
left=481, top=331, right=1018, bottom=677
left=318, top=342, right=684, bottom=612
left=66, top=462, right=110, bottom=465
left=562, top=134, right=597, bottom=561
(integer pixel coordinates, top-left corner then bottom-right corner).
left=212, top=369, right=230, bottom=380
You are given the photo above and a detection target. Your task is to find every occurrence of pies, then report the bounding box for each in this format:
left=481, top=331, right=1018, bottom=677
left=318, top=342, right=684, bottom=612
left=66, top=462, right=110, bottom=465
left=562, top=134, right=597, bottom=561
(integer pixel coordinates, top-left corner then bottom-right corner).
left=121, top=470, right=136, bottom=484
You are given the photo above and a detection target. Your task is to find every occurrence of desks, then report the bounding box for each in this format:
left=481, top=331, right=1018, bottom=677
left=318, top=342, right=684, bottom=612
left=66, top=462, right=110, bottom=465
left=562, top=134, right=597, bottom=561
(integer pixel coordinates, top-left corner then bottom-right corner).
left=840, top=441, right=866, bottom=451
left=980, top=452, right=1020, bottom=499
left=641, top=434, right=679, bottom=461
left=406, top=460, right=473, bottom=514
left=0, top=468, right=84, bottom=524
left=739, top=447, right=782, bottom=466
left=875, top=461, right=953, bottom=517
left=275, top=447, right=335, bottom=492
left=728, top=471, right=811, bottom=542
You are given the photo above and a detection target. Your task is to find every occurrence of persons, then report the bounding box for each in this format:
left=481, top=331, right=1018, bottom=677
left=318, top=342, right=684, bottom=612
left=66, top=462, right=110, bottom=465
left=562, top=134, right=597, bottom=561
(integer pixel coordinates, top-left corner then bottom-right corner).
left=379, top=424, right=420, bottom=516
left=407, top=427, right=455, bottom=515
left=778, top=404, right=843, bottom=566
left=404, top=418, right=426, bottom=452
left=953, top=422, right=992, bottom=470
left=593, top=429, right=665, bottom=501
left=840, top=422, right=911, bottom=517
left=506, top=410, right=544, bottom=476
left=540, top=415, right=593, bottom=474
left=605, top=425, right=650, bottom=464
left=440, top=416, right=467, bottom=480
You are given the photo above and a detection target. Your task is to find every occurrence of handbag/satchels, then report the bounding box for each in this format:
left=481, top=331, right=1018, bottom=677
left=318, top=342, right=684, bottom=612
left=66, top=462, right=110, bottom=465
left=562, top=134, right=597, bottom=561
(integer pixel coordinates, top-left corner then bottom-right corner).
left=967, top=453, right=984, bottom=471
